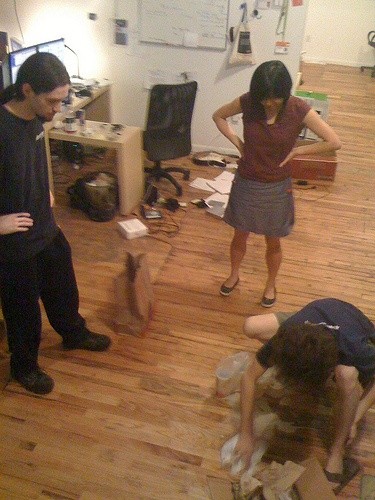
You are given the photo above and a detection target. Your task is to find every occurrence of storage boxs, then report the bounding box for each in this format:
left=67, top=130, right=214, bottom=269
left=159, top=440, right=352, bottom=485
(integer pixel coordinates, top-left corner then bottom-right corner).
left=206, top=457, right=337, bottom=500
left=291, top=139, right=338, bottom=187
left=295, top=90, right=329, bottom=139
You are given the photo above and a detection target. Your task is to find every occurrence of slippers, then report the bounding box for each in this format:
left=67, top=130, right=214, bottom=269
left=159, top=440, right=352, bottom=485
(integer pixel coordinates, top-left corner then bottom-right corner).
left=323, top=456, right=359, bottom=494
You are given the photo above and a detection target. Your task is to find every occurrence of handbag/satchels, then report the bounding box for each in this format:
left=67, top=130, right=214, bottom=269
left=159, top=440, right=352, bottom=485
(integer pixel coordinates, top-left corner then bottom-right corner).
left=113, top=251, right=153, bottom=337
left=69, top=172, right=118, bottom=222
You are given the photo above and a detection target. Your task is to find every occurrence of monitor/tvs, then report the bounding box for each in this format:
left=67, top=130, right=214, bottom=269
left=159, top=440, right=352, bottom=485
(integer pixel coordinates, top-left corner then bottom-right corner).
left=9, top=38, right=65, bottom=86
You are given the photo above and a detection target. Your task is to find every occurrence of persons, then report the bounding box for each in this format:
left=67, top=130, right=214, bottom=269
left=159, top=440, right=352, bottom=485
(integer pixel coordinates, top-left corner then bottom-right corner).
left=211, top=61, right=343, bottom=307
left=234, top=297, right=375, bottom=482
left=0, top=52, right=112, bottom=394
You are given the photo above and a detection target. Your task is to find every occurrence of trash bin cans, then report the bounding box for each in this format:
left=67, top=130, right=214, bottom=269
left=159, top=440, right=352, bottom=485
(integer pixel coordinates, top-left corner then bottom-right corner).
left=82, top=171, right=118, bottom=222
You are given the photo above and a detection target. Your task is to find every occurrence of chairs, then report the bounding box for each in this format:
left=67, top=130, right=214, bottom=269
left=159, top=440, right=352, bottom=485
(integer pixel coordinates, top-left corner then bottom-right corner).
left=143, top=80, right=198, bottom=197
left=360, top=30, right=375, bottom=78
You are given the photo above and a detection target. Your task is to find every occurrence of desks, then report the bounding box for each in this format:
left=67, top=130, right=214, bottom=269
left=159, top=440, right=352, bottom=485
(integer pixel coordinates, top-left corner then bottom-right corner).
left=35, top=83, right=111, bottom=155
left=48, top=119, right=144, bottom=216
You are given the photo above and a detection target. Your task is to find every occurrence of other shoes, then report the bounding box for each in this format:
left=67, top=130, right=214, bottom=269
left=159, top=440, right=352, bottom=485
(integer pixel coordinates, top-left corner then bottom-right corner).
left=261, top=285, right=277, bottom=308
left=220, top=275, right=239, bottom=296
left=64, top=332, right=111, bottom=351
left=11, top=367, right=54, bottom=395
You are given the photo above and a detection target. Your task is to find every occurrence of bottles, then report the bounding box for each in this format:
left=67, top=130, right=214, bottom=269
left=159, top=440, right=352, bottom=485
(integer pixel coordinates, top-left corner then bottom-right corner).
left=64, top=105, right=76, bottom=132
